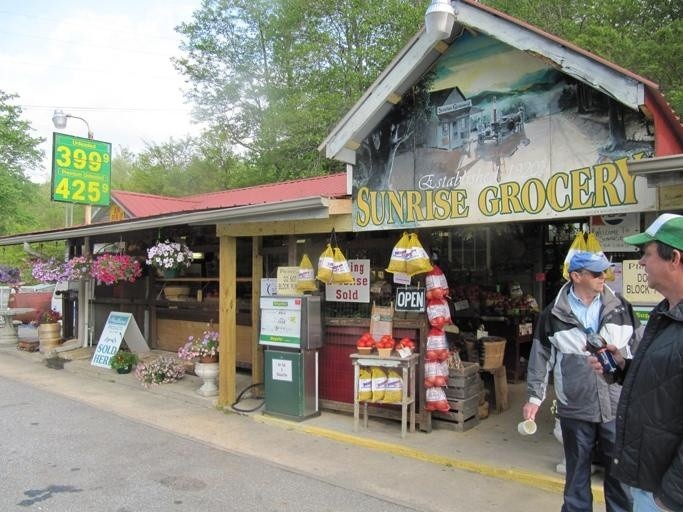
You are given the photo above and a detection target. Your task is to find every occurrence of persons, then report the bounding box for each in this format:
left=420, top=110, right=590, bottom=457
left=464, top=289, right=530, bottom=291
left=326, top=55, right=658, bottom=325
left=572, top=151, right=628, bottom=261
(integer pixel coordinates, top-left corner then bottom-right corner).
left=583, top=211, right=683, bottom=512
left=523, top=251, right=644, bottom=512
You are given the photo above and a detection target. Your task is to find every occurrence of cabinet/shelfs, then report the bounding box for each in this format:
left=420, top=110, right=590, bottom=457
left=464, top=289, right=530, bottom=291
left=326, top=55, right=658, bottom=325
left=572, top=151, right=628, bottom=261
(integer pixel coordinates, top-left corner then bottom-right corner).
left=459, top=309, right=538, bottom=383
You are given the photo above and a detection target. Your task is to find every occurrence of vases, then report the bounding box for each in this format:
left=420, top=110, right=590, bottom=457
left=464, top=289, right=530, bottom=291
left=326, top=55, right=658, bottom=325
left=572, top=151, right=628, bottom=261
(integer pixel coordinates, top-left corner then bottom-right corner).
left=80, top=266, right=87, bottom=272
left=39, top=324, right=62, bottom=352
left=194, top=358, right=219, bottom=396
left=156, top=267, right=176, bottom=278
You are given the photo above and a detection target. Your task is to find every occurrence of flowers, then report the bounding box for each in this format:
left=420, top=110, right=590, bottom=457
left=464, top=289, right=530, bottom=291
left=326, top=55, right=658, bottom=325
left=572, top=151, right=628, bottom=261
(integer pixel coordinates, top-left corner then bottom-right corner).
left=67, top=255, right=92, bottom=268
left=40, top=310, right=62, bottom=324
left=145, top=239, right=193, bottom=270
left=175, top=328, right=220, bottom=361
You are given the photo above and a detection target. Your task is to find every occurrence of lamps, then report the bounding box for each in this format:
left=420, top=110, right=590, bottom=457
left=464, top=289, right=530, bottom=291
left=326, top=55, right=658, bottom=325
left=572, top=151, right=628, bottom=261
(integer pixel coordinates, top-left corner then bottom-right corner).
left=51, top=109, right=95, bottom=140
left=425, top=0, right=456, bottom=40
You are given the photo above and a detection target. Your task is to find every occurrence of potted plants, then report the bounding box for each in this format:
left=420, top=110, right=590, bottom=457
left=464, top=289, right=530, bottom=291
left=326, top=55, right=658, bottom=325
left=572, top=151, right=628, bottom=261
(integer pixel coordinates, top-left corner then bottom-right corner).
left=109, top=350, right=137, bottom=374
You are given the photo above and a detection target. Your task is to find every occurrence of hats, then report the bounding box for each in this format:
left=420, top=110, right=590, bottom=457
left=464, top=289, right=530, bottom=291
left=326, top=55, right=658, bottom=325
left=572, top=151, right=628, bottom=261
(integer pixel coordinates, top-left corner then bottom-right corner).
left=623, top=212, right=683, bottom=251
left=567, top=251, right=616, bottom=273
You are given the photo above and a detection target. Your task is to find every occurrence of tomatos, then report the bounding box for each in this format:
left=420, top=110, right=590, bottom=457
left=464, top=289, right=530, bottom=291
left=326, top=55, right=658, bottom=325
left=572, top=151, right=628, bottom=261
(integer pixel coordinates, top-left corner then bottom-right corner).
left=356, top=332, right=414, bottom=350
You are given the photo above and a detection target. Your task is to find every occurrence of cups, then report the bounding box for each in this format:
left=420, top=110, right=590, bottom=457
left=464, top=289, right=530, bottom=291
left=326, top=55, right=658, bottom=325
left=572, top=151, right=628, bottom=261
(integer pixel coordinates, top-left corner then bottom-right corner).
left=516, top=417, right=539, bottom=437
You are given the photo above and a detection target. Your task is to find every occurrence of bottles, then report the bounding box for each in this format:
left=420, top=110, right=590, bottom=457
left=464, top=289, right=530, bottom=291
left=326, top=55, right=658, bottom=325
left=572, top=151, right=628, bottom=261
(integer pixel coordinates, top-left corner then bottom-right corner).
left=583, top=327, right=622, bottom=387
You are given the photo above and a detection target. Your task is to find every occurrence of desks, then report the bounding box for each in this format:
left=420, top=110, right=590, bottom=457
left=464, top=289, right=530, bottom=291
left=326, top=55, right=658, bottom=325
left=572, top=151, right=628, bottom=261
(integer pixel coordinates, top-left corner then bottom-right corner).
left=349, top=352, right=419, bottom=438
